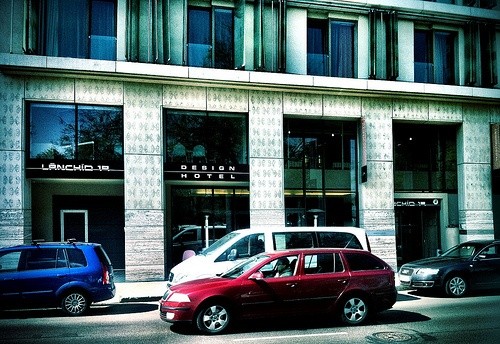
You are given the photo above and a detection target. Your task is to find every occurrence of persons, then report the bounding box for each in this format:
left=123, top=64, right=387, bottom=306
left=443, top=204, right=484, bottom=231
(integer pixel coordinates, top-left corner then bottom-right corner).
left=266, top=258, right=292, bottom=277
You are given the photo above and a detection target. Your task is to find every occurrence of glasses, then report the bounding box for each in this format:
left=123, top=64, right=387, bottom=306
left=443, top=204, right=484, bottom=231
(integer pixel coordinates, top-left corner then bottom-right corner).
left=276, top=263, right=285, bottom=266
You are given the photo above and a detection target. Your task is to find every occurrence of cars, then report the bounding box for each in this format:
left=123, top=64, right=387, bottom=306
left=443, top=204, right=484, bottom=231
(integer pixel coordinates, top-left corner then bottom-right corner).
left=397, top=239, right=500, bottom=298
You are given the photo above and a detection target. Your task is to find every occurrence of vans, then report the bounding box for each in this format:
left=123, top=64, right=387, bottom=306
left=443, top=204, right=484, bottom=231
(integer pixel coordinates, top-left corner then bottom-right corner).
left=166, top=226, right=372, bottom=288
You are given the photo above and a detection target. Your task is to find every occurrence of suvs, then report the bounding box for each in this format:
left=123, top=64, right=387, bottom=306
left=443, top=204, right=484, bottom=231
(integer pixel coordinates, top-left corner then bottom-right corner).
left=0, top=238, right=116, bottom=315
left=159, top=247, right=398, bottom=335
